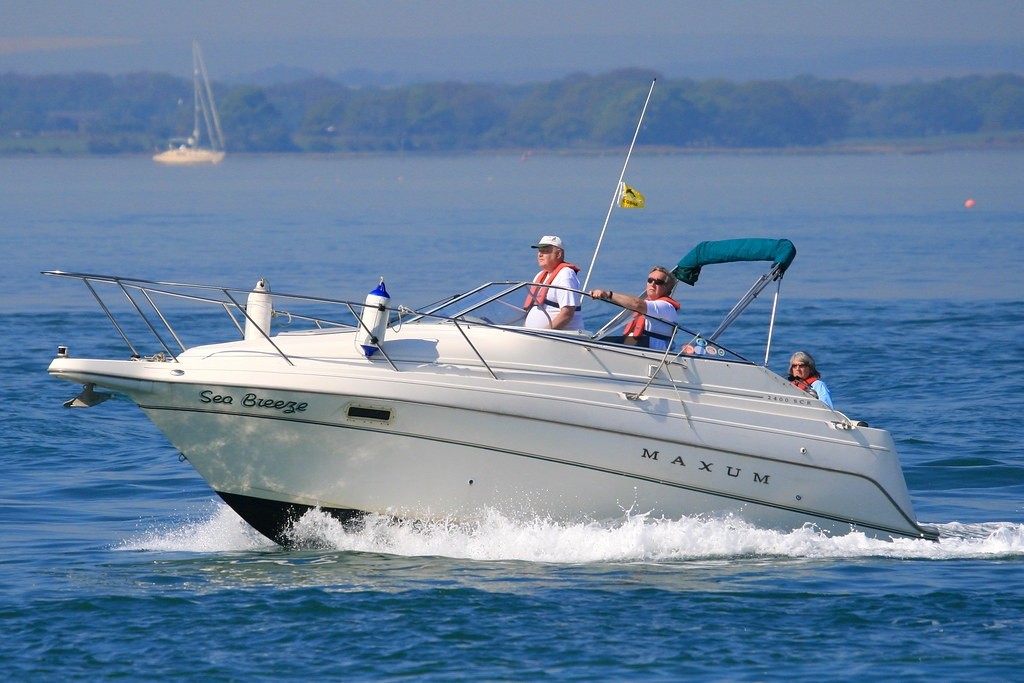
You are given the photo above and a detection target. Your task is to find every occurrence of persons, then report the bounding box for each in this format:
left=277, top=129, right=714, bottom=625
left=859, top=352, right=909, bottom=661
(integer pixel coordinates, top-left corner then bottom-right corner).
left=524, top=236, right=587, bottom=331
left=588, top=265, right=680, bottom=352
left=787, top=351, right=835, bottom=410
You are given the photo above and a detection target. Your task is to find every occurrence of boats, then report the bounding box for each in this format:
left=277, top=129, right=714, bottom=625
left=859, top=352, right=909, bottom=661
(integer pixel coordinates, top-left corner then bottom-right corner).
left=39, top=80, right=943, bottom=551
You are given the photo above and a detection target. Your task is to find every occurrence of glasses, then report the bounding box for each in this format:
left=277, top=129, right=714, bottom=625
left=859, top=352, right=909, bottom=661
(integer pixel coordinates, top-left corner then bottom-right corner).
left=792, top=364, right=807, bottom=369
left=648, top=278, right=664, bottom=285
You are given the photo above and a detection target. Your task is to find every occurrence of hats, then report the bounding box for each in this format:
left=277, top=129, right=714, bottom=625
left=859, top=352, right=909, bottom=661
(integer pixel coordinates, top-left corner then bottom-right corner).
left=531, top=236, right=564, bottom=250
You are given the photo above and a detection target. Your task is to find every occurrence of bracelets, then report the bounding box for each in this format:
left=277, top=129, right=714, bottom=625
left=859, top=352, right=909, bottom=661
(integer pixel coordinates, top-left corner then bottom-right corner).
left=607, top=289, right=613, bottom=299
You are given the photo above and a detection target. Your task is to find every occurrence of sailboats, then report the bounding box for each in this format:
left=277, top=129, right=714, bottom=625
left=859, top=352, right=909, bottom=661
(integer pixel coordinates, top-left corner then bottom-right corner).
left=147, top=36, right=226, bottom=164
